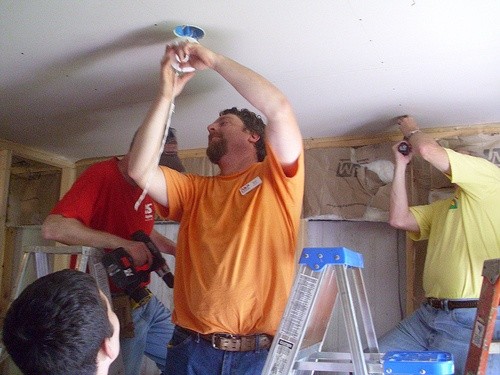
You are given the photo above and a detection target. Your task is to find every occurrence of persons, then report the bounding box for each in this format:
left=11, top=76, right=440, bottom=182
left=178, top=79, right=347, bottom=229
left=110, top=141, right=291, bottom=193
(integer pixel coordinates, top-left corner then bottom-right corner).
left=349, top=115, right=500, bottom=375
left=126, top=42, right=304, bottom=374
left=3, top=269, right=121, bottom=374
left=41, top=126, right=186, bottom=375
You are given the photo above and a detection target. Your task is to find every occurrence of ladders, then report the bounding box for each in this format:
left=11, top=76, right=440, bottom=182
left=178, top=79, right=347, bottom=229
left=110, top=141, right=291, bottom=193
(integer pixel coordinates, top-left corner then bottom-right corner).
left=1, top=245, right=125, bottom=375
left=261, top=247, right=385, bottom=375
left=463, top=260, right=500, bottom=375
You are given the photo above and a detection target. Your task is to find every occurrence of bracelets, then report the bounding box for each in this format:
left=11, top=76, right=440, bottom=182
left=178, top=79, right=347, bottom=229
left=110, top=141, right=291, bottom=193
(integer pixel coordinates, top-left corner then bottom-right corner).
left=406, top=130, right=420, bottom=138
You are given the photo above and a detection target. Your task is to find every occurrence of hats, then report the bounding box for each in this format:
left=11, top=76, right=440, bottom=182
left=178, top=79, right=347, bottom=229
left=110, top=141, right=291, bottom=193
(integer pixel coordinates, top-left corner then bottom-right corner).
left=130, top=127, right=185, bottom=172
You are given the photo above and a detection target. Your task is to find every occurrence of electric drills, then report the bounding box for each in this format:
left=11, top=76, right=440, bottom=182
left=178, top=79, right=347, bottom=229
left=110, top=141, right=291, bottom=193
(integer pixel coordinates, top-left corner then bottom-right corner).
left=103, top=230, right=176, bottom=294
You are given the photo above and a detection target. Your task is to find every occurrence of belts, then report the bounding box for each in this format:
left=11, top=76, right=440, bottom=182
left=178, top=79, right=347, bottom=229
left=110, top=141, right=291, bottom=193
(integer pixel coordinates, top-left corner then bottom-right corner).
left=176, top=326, right=271, bottom=352
left=427, top=298, right=478, bottom=309
